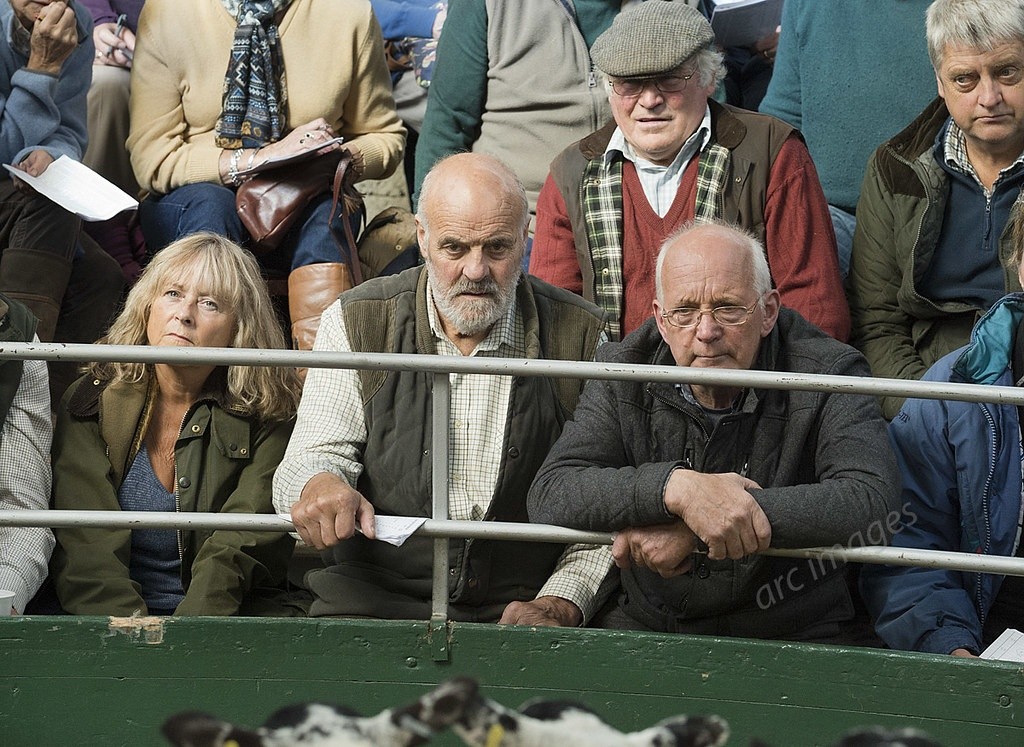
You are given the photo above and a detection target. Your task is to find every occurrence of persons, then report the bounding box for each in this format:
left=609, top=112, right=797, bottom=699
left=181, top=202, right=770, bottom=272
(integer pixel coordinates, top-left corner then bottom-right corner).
left=52, top=232, right=302, bottom=617
left=272, top=152, right=619, bottom=628
left=526, top=222, right=902, bottom=646
left=0, top=292, right=51, bottom=617
left=851, top=189, right=1024, bottom=657
left=0, top=0, right=1024, bottom=421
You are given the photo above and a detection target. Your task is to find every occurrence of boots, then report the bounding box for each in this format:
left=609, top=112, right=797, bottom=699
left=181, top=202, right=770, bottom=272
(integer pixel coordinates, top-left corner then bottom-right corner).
left=287, top=262, right=353, bottom=411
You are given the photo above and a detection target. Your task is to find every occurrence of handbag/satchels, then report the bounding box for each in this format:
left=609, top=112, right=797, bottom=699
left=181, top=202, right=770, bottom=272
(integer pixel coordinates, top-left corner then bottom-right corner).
left=234, top=145, right=345, bottom=248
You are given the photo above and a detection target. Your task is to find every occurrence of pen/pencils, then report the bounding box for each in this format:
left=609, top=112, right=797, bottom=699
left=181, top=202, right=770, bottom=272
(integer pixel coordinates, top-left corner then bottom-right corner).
left=35, top=16, right=81, bottom=48
left=107, top=14, right=128, bottom=58
left=317, top=123, right=331, bottom=130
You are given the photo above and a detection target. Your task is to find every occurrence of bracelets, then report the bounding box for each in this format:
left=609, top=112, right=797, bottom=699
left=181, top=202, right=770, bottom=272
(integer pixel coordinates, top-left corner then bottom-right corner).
left=228, top=149, right=245, bottom=187
left=246, top=147, right=260, bottom=179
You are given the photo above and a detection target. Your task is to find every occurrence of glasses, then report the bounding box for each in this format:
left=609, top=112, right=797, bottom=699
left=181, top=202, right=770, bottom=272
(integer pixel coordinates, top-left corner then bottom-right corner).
left=660, top=290, right=766, bottom=329
left=606, top=64, right=701, bottom=97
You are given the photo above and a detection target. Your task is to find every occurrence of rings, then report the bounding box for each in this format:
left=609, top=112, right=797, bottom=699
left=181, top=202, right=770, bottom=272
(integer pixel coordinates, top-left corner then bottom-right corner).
left=763, top=50, right=769, bottom=59
left=96, top=51, right=103, bottom=58
left=299, top=139, right=305, bottom=144
left=304, top=132, right=314, bottom=138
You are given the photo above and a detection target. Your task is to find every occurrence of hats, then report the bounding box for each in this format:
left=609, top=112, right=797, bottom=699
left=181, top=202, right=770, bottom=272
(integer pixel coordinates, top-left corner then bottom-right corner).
left=590, top=0, right=716, bottom=80
